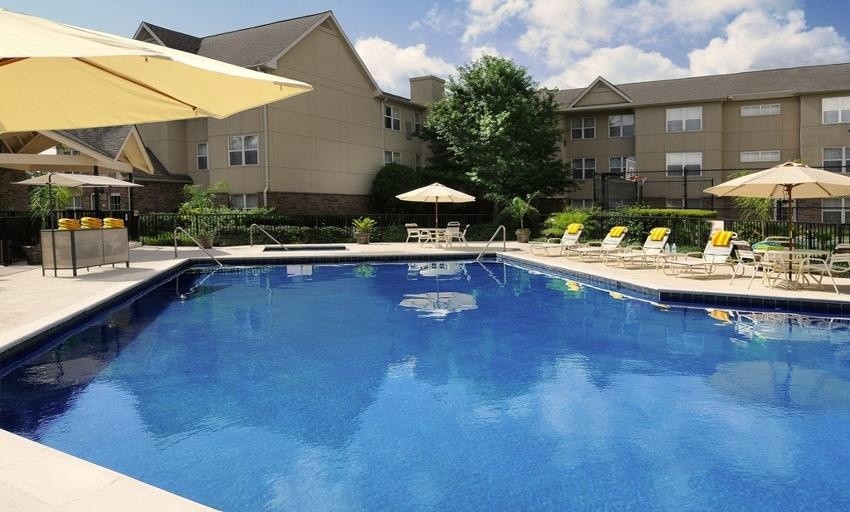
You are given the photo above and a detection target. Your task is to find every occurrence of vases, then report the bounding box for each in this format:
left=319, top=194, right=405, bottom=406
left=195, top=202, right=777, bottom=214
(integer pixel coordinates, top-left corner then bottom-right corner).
left=20, top=243, right=43, bottom=265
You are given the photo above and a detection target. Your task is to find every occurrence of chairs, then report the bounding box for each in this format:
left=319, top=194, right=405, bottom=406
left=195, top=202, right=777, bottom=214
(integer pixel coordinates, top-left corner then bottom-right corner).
left=762, top=235, right=798, bottom=285
left=563, top=226, right=628, bottom=262
left=789, top=243, right=850, bottom=295
left=729, top=240, right=776, bottom=289
left=529, top=223, right=584, bottom=257
left=662, top=231, right=738, bottom=276
left=402, top=220, right=472, bottom=252
left=603, top=228, right=670, bottom=267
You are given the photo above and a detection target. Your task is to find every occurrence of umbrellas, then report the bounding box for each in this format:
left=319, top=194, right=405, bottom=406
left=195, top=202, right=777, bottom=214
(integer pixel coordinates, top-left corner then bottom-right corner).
left=395, top=182, right=476, bottom=243
left=0, top=8, right=315, bottom=135
left=702, top=162, right=850, bottom=281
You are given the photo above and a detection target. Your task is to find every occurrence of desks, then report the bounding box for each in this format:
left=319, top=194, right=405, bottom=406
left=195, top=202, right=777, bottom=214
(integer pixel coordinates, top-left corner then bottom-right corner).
left=563, top=243, right=582, bottom=257
left=754, top=248, right=830, bottom=291
left=656, top=253, right=687, bottom=271
left=39, top=227, right=130, bottom=276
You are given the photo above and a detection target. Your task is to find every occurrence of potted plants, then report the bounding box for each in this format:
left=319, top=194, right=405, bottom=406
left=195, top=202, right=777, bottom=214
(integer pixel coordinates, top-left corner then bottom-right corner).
left=176, top=177, right=231, bottom=249
left=484, top=188, right=541, bottom=243
left=351, top=215, right=378, bottom=244
